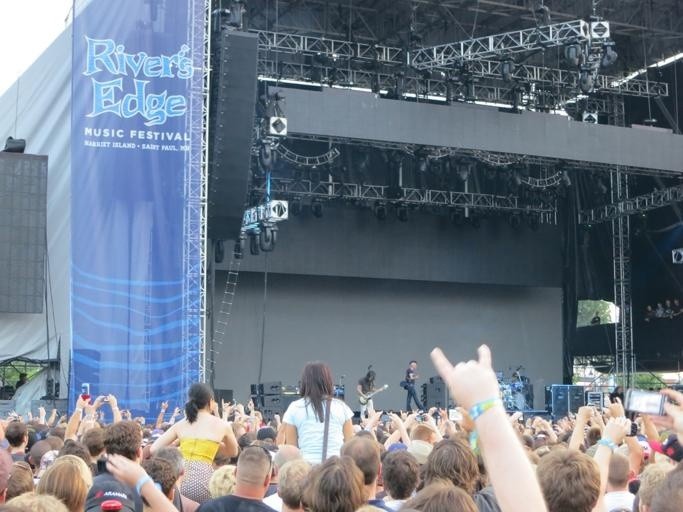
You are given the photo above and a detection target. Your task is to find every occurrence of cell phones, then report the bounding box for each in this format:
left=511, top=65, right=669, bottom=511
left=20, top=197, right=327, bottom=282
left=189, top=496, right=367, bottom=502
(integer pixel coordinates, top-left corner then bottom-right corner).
left=80, top=382, right=90, bottom=400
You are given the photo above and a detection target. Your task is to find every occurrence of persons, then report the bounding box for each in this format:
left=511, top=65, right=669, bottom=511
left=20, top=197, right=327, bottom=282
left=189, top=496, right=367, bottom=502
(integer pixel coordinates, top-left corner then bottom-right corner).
left=608, top=385, right=626, bottom=404
left=402, top=360, right=425, bottom=408
left=642, top=300, right=682, bottom=322
left=147, top=385, right=242, bottom=465
left=353, top=372, right=378, bottom=421
left=591, top=312, right=601, bottom=325
left=430, top=340, right=548, bottom=512
left=0, top=390, right=683, bottom=512
left=277, top=363, right=356, bottom=464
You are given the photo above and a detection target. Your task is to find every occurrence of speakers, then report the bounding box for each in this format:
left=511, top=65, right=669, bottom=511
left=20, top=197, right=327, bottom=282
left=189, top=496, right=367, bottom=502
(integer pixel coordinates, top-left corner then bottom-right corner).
left=250, top=380, right=283, bottom=424
left=214, top=388, right=234, bottom=421
left=209, top=29, right=261, bottom=241
left=422, top=375, right=449, bottom=410
left=545, top=385, right=604, bottom=423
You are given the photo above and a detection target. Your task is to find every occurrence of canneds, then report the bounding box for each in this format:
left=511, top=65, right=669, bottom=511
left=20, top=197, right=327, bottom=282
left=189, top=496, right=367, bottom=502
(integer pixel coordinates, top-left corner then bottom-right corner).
left=82, top=383, right=89, bottom=395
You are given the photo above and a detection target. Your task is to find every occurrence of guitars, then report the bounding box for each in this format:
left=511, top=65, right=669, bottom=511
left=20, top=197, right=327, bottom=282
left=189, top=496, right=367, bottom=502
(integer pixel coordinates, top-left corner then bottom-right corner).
left=358, top=384, right=389, bottom=404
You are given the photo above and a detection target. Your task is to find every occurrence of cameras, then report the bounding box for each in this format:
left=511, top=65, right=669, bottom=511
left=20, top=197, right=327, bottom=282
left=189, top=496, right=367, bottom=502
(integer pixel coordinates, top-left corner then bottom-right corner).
left=626, top=422, right=638, bottom=437
left=97, top=459, right=110, bottom=474
left=624, top=388, right=670, bottom=416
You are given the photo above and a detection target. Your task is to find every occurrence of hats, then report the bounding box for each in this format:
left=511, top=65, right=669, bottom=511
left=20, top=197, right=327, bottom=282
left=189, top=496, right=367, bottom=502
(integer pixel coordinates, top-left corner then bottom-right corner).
left=84, top=481, right=135, bottom=512
